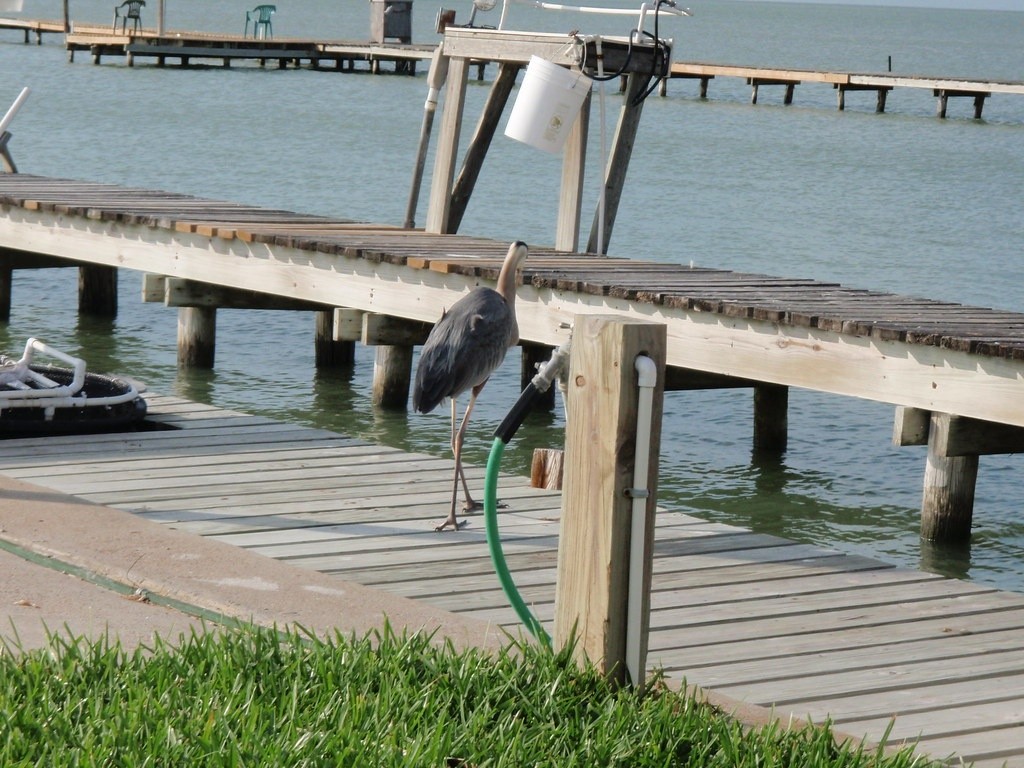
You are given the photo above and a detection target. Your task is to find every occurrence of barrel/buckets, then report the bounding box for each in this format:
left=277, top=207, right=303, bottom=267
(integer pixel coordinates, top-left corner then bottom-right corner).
left=503, top=35, right=593, bottom=155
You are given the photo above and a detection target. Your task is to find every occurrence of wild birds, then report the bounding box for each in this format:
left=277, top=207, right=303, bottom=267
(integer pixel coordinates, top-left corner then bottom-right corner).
left=412, top=239, right=531, bottom=533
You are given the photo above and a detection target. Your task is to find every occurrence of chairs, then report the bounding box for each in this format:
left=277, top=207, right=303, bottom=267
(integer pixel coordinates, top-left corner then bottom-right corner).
left=113, top=0, right=146, bottom=36
left=244, top=4, right=276, bottom=40
